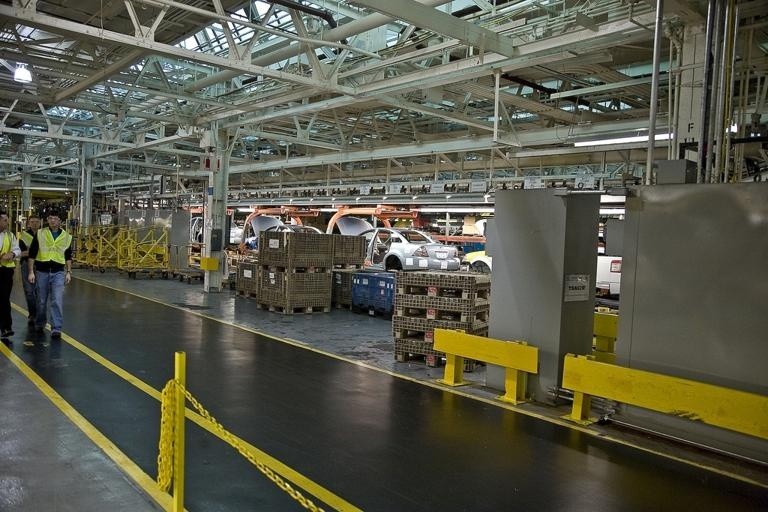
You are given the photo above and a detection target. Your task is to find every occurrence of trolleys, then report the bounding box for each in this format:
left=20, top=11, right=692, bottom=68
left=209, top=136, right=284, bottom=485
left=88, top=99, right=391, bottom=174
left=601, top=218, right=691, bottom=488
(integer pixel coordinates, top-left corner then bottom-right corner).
left=70, top=225, right=203, bottom=284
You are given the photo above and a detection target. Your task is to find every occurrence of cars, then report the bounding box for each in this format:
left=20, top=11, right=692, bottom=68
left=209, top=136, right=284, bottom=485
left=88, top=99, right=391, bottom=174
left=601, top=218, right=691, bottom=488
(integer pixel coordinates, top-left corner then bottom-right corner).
left=596, top=254, right=623, bottom=296
left=245, top=216, right=327, bottom=250
left=336, top=217, right=464, bottom=271
left=462, top=251, right=492, bottom=273
left=191, top=217, right=242, bottom=245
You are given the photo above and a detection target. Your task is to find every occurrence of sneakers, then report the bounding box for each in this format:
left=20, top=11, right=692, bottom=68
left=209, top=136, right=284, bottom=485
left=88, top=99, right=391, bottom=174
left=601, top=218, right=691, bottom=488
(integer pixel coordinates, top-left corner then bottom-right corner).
left=51, top=332, right=60, bottom=338
left=0, top=328, right=15, bottom=337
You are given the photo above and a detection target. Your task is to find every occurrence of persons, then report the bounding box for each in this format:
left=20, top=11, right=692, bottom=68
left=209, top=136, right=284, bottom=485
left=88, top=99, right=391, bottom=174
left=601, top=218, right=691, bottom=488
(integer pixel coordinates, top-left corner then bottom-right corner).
left=27, top=210, right=72, bottom=338
left=19, top=215, right=41, bottom=330
left=0, top=211, right=21, bottom=337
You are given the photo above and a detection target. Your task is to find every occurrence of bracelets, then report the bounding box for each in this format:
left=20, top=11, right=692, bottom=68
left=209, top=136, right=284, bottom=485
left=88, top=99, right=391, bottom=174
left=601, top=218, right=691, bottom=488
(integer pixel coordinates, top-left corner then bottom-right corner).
left=67, top=270, right=72, bottom=273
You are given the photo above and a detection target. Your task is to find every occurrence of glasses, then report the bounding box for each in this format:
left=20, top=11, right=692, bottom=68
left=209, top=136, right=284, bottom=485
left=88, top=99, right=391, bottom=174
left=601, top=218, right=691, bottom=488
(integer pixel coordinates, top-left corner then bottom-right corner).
left=47, top=216, right=58, bottom=221
